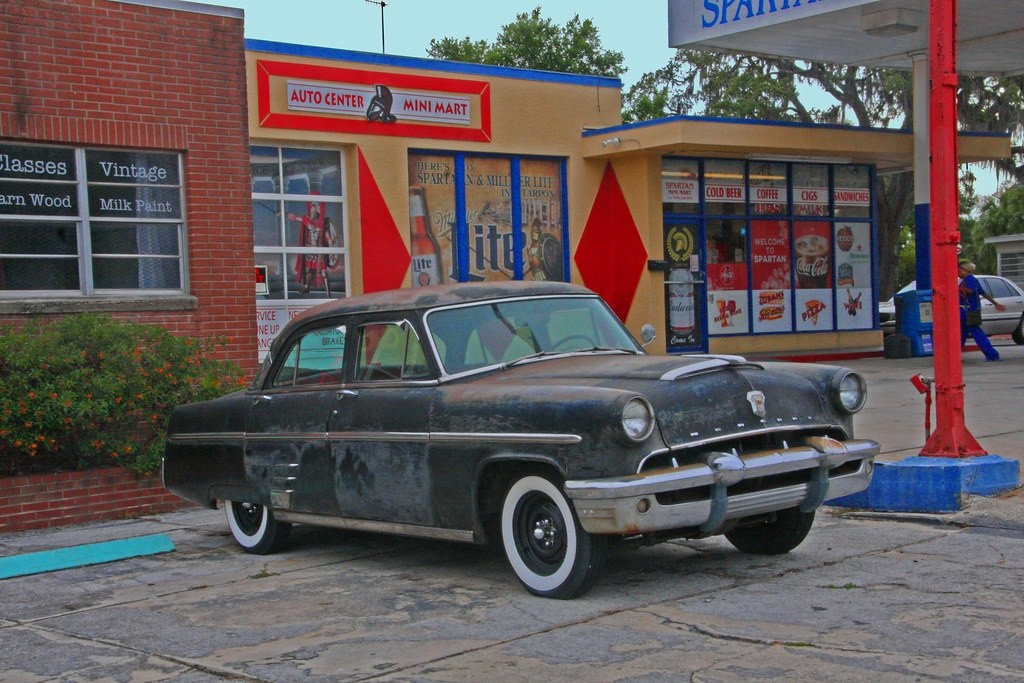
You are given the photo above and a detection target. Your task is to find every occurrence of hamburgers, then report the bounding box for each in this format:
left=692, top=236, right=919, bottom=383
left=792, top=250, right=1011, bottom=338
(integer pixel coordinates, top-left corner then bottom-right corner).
left=758, top=291, right=784, bottom=319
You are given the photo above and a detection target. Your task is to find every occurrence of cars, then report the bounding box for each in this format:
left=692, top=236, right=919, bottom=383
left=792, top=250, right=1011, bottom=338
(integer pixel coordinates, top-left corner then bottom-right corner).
left=159, top=279, right=882, bottom=602
left=877, top=274, right=1024, bottom=347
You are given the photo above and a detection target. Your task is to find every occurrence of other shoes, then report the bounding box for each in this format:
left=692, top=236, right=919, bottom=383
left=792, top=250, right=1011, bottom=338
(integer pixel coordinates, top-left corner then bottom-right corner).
left=984, top=356, right=1000, bottom=362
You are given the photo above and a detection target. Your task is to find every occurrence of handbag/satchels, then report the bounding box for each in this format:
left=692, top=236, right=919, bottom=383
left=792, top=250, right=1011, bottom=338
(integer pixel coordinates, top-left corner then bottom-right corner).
left=964, top=310, right=983, bottom=328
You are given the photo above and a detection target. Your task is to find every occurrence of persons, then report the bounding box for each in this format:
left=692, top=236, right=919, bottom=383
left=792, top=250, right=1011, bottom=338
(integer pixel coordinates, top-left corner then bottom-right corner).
left=275, top=200, right=336, bottom=298
left=957, top=261, right=1007, bottom=361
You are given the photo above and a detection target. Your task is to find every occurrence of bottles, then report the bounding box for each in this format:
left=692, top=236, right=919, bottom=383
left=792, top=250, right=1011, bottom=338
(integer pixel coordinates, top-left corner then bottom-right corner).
left=846, top=289, right=862, bottom=315
left=409, top=185, right=444, bottom=288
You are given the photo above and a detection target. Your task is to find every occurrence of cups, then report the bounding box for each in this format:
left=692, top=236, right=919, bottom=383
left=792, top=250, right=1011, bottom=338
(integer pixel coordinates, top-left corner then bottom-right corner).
left=716, top=298, right=736, bottom=328
left=795, top=233, right=829, bottom=288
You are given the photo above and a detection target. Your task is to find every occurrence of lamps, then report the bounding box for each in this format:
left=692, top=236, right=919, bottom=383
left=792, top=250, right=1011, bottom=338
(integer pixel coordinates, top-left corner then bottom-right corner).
left=861, top=8, right=924, bottom=40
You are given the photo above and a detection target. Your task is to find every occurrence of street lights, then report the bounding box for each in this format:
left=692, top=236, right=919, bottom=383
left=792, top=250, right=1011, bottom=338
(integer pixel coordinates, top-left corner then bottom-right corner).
left=365, top=0, right=390, bottom=54
